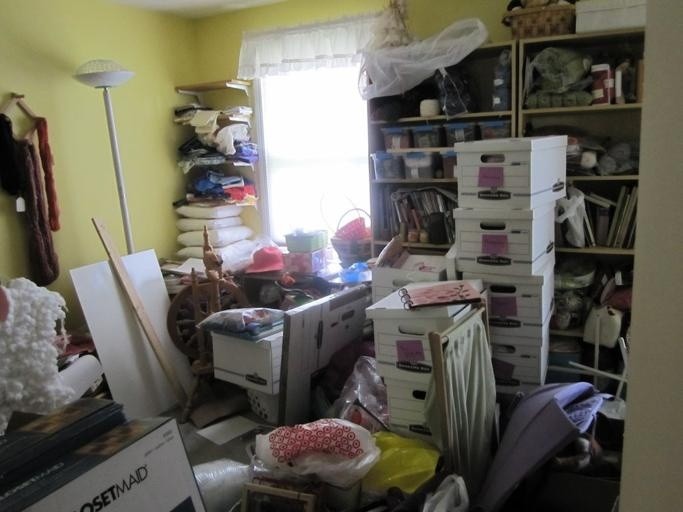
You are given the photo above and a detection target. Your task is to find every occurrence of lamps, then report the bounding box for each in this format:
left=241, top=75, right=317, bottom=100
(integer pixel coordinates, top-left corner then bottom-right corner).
left=73, top=57, right=136, bottom=254
left=582, top=306, right=628, bottom=422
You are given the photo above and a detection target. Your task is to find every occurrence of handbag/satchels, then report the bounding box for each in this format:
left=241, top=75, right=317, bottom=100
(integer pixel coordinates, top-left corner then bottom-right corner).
left=555, top=187, right=585, bottom=248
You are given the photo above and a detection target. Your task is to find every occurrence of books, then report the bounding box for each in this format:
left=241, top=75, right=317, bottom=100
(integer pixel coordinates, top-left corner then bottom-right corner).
left=398, top=281, right=481, bottom=310
left=584, top=185, right=638, bottom=249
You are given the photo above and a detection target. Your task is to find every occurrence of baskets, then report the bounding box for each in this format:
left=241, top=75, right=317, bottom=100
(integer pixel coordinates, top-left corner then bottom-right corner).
left=329, top=208, right=372, bottom=263
left=502, top=5, right=575, bottom=38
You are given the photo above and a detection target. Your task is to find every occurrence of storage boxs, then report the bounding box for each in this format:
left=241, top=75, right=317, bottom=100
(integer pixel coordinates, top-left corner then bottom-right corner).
left=575, top=2, right=645, bottom=34
left=209, top=322, right=283, bottom=394
left=283, top=228, right=328, bottom=274
left=369, top=364, right=548, bottom=442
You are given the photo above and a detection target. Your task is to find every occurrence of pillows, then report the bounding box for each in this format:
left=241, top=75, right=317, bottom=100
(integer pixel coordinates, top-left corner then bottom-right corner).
left=176, top=206, right=258, bottom=271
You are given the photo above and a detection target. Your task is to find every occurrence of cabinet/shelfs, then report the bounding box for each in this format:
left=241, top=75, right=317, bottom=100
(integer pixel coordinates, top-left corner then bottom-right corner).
left=169, top=74, right=256, bottom=205
left=366, top=29, right=644, bottom=375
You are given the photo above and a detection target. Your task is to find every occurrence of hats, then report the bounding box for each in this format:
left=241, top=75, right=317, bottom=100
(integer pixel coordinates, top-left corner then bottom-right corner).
left=244, top=246, right=283, bottom=273
